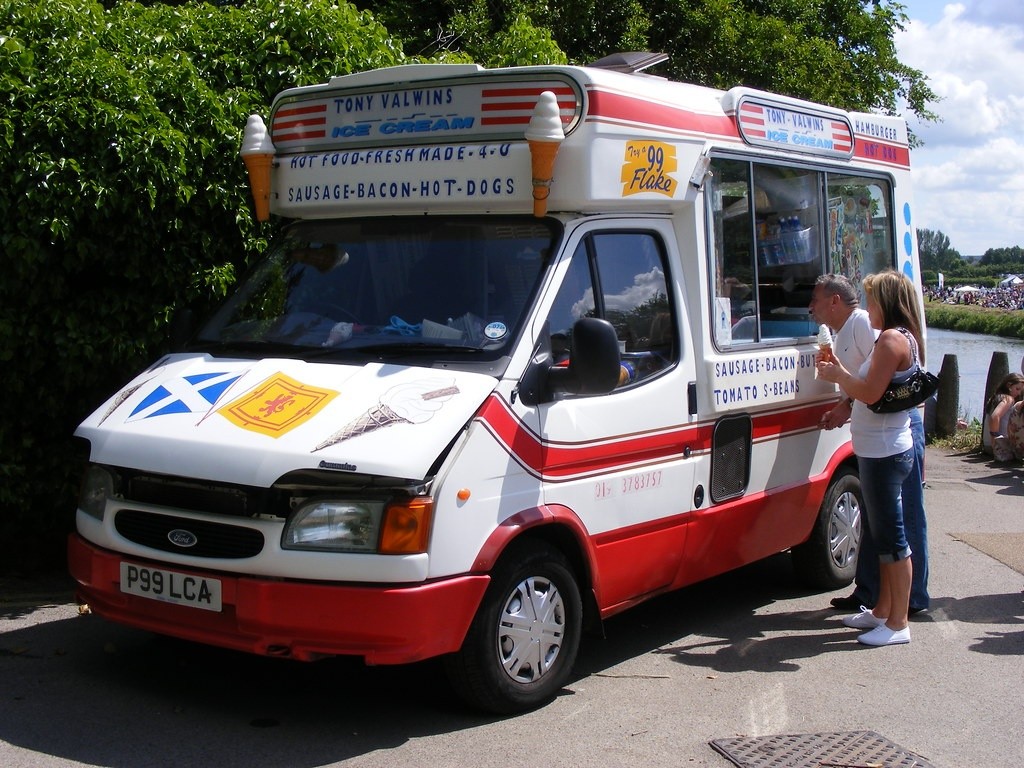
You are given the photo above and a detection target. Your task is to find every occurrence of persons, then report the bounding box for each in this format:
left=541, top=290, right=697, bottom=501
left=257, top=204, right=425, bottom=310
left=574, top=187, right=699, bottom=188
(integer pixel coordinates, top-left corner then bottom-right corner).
left=922, top=284, right=1024, bottom=311
left=810, top=272, right=930, bottom=646
left=983, top=372, right=1024, bottom=465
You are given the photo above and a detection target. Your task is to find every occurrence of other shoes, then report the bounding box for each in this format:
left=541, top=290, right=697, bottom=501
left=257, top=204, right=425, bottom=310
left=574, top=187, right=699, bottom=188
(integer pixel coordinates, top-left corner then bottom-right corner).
left=908, top=606, right=925, bottom=616
left=830, top=593, right=876, bottom=611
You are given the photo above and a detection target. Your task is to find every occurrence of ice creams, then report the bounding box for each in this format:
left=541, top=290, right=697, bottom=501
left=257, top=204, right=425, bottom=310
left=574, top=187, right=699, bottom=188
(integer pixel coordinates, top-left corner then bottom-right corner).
left=239, top=113, right=276, bottom=221
left=817, top=323, right=834, bottom=364
left=310, top=376, right=457, bottom=449
left=98, top=356, right=171, bottom=426
left=524, top=91, right=565, bottom=215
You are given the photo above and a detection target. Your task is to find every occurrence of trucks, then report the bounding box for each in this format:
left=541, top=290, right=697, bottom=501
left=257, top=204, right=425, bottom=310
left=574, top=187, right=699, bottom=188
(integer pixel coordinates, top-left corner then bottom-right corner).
left=68, top=50, right=925, bottom=709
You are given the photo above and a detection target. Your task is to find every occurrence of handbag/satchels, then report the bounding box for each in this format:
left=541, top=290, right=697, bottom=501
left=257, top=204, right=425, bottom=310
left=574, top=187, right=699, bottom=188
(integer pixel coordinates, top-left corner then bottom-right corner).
left=993, top=434, right=1012, bottom=461
left=868, top=324, right=941, bottom=414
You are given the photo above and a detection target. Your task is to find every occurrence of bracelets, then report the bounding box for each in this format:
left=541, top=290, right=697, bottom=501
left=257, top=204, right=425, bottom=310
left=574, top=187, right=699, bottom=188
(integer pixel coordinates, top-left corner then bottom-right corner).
left=990, top=432, right=1000, bottom=437
left=848, top=398, right=854, bottom=413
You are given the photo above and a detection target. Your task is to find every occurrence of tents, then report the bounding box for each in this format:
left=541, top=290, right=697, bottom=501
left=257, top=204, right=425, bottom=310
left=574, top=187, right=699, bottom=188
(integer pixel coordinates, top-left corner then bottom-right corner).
left=955, top=286, right=980, bottom=292
left=1001, top=275, right=1023, bottom=287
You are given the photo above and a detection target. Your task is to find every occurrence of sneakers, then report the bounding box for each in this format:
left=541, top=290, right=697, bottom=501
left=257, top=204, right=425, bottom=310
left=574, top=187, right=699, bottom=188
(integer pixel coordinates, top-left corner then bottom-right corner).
left=842, top=605, right=889, bottom=628
left=857, top=621, right=911, bottom=646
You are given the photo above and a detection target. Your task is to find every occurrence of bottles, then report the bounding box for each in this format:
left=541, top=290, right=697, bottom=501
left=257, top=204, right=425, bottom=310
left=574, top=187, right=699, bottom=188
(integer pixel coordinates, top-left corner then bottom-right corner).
left=779, top=216, right=808, bottom=260
